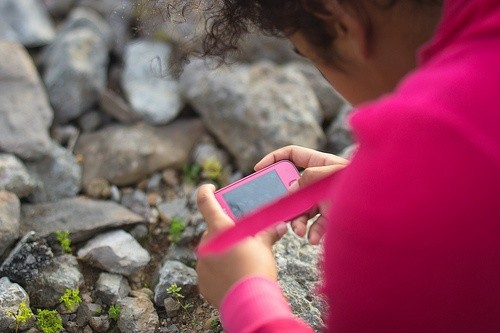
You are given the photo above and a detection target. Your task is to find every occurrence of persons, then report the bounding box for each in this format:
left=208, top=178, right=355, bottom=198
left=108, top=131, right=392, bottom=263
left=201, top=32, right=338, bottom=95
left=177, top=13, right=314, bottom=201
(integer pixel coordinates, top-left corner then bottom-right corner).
left=192, top=0, right=499, bottom=332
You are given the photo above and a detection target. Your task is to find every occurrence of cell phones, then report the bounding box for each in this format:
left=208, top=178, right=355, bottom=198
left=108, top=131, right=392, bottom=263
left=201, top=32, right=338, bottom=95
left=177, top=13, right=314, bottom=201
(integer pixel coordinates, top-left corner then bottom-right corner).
left=213, top=160, right=303, bottom=225
